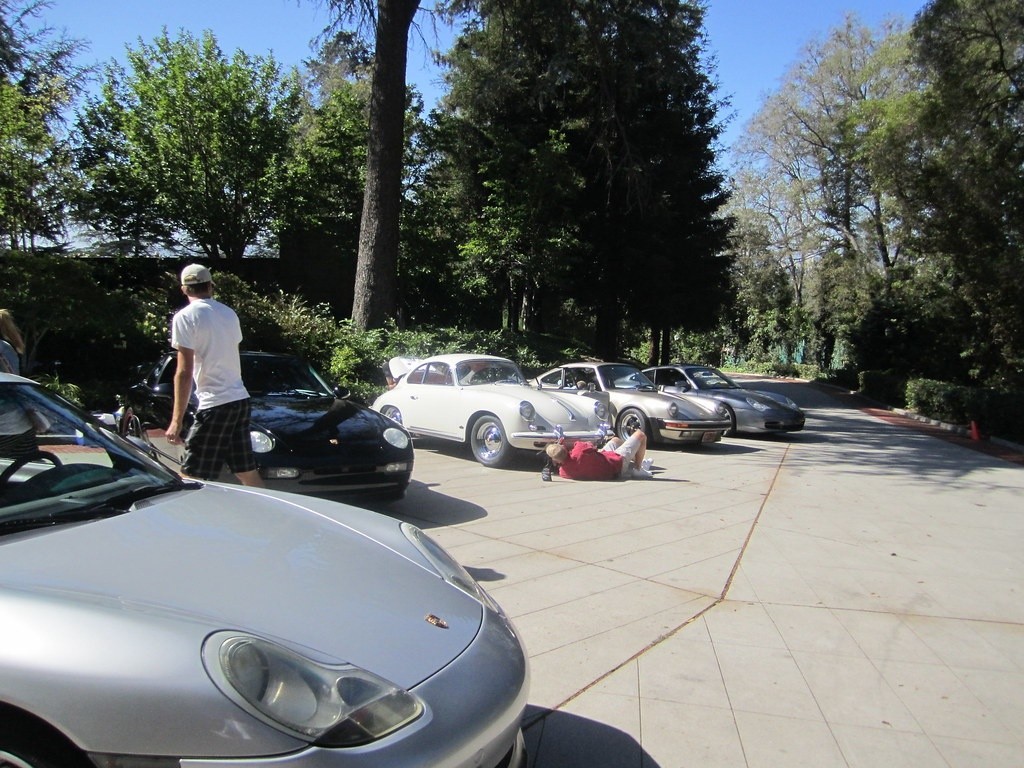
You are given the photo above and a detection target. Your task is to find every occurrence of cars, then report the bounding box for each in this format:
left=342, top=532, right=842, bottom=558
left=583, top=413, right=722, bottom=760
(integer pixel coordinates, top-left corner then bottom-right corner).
left=613, top=364, right=805, bottom=433
left=366, top=353, right=615, bottom=468
left=124, top=350, right=415, bottom=505
left=0, top=371, right=531, bottom=768
left=495, top=362, right=733, bottom=445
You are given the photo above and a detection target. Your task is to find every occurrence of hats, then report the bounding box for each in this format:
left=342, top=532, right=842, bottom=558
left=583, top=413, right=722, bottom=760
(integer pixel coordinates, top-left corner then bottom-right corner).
left=181, top=263, right=216, bottom=286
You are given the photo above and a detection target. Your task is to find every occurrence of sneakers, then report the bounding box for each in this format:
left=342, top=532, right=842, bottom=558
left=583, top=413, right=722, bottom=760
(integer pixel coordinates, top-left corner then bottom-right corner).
left=632, top=466, right=652, bottom=477
left=641, top=458, right=654, bottom=471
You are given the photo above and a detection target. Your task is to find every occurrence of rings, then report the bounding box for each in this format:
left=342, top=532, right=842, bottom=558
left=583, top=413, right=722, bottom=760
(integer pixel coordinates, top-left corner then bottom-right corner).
left=170, top=440, right=173, bottom=442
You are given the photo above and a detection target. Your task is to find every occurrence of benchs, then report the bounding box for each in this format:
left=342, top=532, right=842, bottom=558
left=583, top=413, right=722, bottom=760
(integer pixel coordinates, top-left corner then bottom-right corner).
left=408, top=372, right=451, bottom=384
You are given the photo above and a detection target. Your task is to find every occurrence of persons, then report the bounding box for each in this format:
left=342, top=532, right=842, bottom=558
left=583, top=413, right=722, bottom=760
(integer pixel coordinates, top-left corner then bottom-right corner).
left=0, top=309, right=49, bottom=463
left=545, top=430, right=654, bottom=481
left=382, top=363, right=397, bottom=389
left=165, top=263, right=265, bottom=488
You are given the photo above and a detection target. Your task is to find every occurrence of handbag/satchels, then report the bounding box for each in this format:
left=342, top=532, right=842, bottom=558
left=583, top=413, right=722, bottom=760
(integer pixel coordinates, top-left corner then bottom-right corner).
left=23, top=404, right=50, bottom=432
